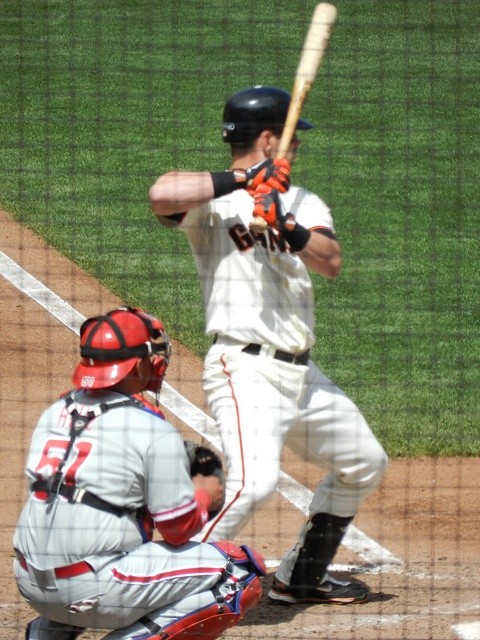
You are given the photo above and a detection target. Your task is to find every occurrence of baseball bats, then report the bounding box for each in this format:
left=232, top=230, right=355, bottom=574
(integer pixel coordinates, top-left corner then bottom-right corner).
left=248, top=1, right=339, bottom=233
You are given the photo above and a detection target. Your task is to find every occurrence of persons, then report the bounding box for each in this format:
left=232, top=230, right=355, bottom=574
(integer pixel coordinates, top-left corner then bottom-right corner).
left=11, top=305, right=267, bottom=640
left=147, top=87, right=388, bottom=606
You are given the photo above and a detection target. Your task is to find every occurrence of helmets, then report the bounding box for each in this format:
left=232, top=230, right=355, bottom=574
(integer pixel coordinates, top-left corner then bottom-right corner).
left=222, top=85, right=313, bottom=143
left=72, top=305, right=172, bottom=406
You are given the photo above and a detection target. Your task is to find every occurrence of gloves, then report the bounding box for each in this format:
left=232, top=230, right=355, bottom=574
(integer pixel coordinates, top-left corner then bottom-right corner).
left=231, top=157, right=293, bottom=194
left=249, top=184, right=297, bottom=237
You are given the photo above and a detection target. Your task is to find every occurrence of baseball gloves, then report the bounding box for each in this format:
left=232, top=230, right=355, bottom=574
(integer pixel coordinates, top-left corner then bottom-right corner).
left=184, top=441, right=225, bottom=521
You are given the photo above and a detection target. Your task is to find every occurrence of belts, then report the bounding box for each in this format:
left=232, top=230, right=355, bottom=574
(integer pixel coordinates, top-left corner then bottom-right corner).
left=17, top=552, right=94, bottom=580
left=212, top=333, right=310, bottom=366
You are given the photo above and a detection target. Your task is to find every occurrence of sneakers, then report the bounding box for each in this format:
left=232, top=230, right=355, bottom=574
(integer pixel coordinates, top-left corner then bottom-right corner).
left=268, top=574, right=371, bottom=604
